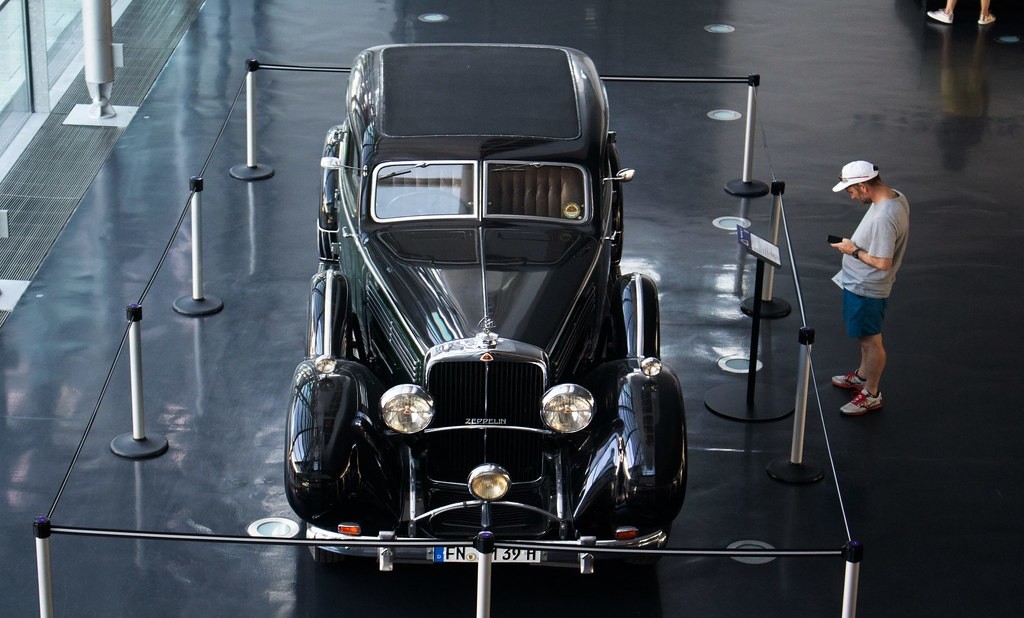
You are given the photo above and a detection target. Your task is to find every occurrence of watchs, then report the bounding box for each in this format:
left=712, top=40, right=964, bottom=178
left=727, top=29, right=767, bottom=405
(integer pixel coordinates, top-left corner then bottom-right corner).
left=852, top=248, right=862, bottom=259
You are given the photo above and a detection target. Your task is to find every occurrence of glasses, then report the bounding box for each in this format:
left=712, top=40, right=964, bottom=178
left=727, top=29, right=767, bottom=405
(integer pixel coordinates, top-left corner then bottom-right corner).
left=839, top=174, right=869, bottom=181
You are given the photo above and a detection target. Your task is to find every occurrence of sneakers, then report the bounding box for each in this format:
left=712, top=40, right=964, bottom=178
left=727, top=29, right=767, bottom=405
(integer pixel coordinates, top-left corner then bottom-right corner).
left=832, top=368, right=866, bottom=391
left=927, top=9, right=953, bottom=23
left=840, top=388, right=883, bottom=415
left=978, top=14, right=995, bottom=24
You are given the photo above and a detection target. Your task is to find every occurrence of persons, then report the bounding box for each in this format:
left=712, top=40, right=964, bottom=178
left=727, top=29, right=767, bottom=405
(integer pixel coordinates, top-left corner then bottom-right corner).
left=829, top=160, right=910, bottom=415
left=926, top=0, right=996, bottom=25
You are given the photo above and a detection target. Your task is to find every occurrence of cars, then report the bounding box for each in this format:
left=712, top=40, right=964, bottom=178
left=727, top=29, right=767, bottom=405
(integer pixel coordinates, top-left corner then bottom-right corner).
left=280, top=42, right=688, bottom=569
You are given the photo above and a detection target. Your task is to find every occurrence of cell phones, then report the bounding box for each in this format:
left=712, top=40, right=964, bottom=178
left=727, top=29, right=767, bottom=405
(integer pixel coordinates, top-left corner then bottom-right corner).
left=827, top=234, right=843, bottom=244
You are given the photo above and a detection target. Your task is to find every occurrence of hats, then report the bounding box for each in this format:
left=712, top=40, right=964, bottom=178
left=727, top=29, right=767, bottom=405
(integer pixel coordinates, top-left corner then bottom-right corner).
left=832, top=160, right=879, bottom=193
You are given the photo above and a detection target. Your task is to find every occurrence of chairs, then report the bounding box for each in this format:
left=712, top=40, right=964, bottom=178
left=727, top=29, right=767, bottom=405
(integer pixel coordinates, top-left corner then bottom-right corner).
left=379, top=165, right=584, bottom=221
